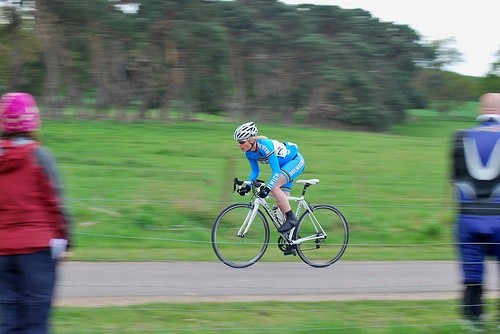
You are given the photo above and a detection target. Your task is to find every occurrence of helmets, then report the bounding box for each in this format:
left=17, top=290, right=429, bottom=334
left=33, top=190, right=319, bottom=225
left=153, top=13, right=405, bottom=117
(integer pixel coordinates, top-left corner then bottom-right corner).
left=0, top=93, right=40, bottom=131
left=234, top=122, right=258, bottom=140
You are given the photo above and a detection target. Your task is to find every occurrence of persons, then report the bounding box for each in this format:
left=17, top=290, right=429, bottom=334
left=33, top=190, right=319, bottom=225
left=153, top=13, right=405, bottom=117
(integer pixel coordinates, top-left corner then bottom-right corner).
left=448, top=92, right=500, bottom=321
left=233, top=122, right=305, bottom=256
left=0, top=92, right=72, bottom=334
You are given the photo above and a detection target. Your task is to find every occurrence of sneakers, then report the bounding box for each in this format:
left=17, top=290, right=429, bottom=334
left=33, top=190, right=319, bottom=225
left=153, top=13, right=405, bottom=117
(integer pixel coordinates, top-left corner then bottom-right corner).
left=463, top=289, right=483, bottom=321
left=284, top=235, right=302, bottom=255
left=277, top=214, right=298, bottom=232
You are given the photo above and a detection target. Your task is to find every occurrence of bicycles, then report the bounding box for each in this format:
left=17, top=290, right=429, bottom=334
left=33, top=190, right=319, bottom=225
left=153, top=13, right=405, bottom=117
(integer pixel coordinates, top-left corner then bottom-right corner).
left=210, top=177, right=354, bottom=268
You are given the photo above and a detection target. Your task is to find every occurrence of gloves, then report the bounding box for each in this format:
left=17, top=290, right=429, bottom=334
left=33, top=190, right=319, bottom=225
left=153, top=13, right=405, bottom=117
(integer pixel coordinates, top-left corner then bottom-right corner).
left=237, top=185, right=250, bottom=196
left=257, top=186, right=270, bottom=198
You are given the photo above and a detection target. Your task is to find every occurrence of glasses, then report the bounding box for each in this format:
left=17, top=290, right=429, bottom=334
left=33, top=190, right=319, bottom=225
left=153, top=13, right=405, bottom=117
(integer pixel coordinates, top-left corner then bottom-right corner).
left=238, top=140, right=248, bottom=144
